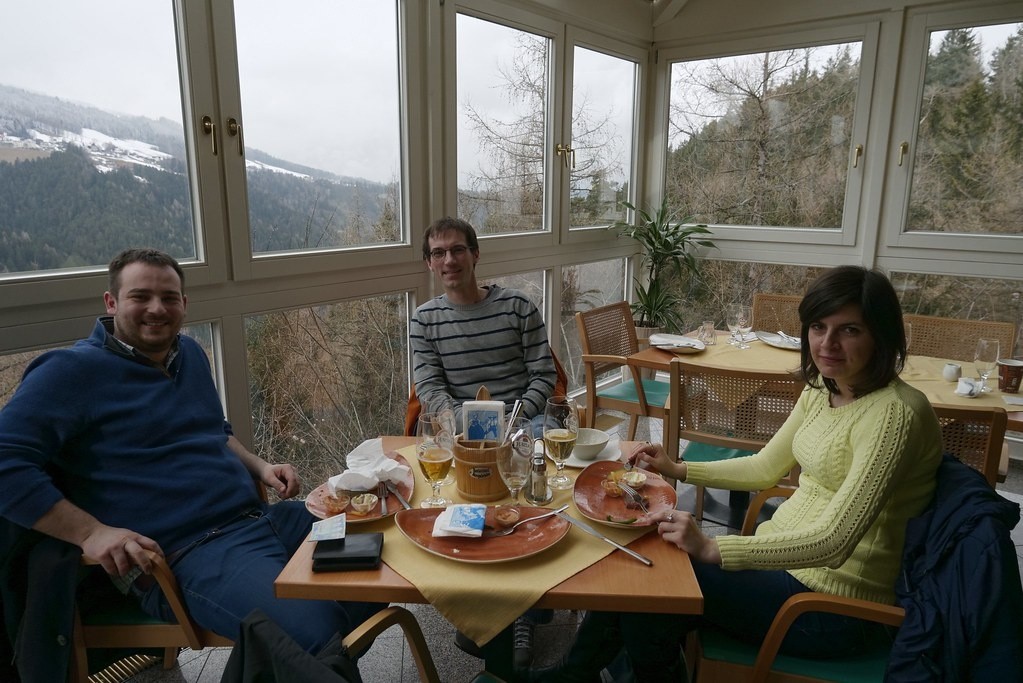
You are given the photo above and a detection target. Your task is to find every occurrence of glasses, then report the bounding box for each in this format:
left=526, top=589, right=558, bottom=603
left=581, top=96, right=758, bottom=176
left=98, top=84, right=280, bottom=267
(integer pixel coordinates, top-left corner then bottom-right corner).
left=428, top=245, right=476, bottom=261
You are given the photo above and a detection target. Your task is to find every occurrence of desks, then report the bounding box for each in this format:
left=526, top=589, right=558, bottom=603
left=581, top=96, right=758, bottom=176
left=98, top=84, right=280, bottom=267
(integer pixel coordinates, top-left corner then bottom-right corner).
left=274, top=435, right=703, bottom=683
left=626, top=327, right=1023, bottom=489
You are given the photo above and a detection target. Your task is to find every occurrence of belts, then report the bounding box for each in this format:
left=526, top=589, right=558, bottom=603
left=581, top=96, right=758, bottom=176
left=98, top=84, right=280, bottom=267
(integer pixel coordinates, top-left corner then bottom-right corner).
left=127, top=549, right=183, bottom=606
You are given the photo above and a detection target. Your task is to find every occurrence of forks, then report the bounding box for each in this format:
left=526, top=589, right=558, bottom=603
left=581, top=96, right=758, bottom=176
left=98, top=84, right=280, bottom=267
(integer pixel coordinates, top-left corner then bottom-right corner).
left=489, top=504, right=571, bottom=537
left=377, top=483, right=391, bottom=517
left=777, top=331, right=798, bottom=348
left=617, top=482, right=661, bottom=527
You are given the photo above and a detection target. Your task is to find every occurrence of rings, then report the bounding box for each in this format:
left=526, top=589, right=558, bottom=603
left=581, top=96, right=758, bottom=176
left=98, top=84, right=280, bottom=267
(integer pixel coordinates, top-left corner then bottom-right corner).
left=667, top=513, right=673, bottom=522
left=646, top=441, right=650, bottom=443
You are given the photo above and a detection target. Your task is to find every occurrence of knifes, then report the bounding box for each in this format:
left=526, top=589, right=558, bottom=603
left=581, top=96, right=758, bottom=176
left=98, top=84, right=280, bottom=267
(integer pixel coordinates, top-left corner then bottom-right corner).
left=552, top=511, right=652, bottom=567
left=382, top=480, right=411, bottom=510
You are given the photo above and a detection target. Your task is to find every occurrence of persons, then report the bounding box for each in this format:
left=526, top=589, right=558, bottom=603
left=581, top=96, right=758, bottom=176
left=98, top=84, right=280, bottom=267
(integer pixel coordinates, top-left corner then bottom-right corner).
left=516, top=266, right=945, bottom=683
left=0, top=248, right=389, bottom=683
left=410, top=217, right=561, bottom=658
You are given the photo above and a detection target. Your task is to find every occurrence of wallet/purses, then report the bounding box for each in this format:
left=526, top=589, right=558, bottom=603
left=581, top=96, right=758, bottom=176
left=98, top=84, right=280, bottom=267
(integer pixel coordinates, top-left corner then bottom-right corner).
left=311, top=532, right=384, bottom=572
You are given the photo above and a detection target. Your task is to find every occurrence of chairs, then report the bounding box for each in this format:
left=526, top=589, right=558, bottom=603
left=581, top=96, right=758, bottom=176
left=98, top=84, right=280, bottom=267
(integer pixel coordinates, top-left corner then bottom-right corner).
left=734, top=293, right=803, bottom=443
left=240, top=606, right=509, bottom=683
left=931, top=404, right=1007, bottom=490
left=666, top=357, right=805, bottom=499
left=70, top=479, right=269, bottom=682
left=685, top=488, right=905, bottom=683
left=903, top=314, right=1016, bottom=484
left=575, top=301, right=707, bottom=481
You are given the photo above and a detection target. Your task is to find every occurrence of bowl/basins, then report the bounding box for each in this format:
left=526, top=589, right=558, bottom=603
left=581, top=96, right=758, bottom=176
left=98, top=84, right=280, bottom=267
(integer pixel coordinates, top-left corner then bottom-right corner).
left=572, top=428, right=610, bottom=460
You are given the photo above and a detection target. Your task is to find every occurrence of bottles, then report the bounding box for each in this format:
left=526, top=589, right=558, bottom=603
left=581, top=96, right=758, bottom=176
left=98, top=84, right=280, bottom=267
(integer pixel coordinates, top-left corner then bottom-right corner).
left=698, top=320, right=717, bottom=345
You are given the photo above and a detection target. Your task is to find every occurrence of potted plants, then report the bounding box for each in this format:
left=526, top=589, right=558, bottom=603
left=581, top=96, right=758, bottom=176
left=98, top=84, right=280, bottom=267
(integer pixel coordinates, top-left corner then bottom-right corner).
left=608, top=201, right=719, bottom=383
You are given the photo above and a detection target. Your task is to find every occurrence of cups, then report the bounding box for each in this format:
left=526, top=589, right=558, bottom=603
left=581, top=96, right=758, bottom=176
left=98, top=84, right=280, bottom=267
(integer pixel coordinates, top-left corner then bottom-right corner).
left=942, top=362, right=963, bottom=381
left=493, top=504, right=521, bottom=524
left=996, top=359, right=1023, bottom=394
left=321, top=494, right=350, bottom=513
left=351, top=492, right=378, bottom=513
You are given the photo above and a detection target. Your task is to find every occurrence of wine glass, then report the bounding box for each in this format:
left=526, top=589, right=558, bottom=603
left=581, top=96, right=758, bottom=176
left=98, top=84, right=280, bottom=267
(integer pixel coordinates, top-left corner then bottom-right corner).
left=496, top=416, right=534, bottom=505
left=974, top=338, right=1000, bottom=392
left=736, top=306, right=753, bottom=349
left=896, top=321, right=912, bottom=373
left=725, top=303, right=743, bottom=344
left=542, top=395, right=579, bottom=490
left=415, top=412, right=455, bottom=508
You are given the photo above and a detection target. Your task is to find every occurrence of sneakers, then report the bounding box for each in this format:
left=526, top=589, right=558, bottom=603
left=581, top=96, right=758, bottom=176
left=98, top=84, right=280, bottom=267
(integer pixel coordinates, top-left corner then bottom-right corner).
left=514, top=616, right=535, bottom=669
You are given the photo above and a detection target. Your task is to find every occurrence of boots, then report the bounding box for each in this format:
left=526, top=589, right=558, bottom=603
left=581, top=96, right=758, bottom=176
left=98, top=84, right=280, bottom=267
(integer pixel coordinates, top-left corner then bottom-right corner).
left=513, top=610, right=625, bottom=683
left=628, top=645, right=685, bottom=683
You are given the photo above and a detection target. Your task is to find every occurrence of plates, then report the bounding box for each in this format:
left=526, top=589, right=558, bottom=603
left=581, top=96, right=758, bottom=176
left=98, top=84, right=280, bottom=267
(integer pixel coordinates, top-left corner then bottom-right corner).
left=755, top=331, right=800, bottom=350
left=562, top=441, right=621, bottom=468
left=426, top=432, right=462, bottom=468
left=305, top=452, right=415, bottom=523
left=648, top=333, right=705, bottom=353
left=572, top=460, right=678, bottom=526
left=394, top=506, right=571, bottom=568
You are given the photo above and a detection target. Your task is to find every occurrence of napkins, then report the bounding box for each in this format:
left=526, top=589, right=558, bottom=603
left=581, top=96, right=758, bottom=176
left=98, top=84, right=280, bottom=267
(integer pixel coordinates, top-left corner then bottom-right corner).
left=327, top=438, right=409, bottom=497
left=432, top=503, right=488, bottom=537
